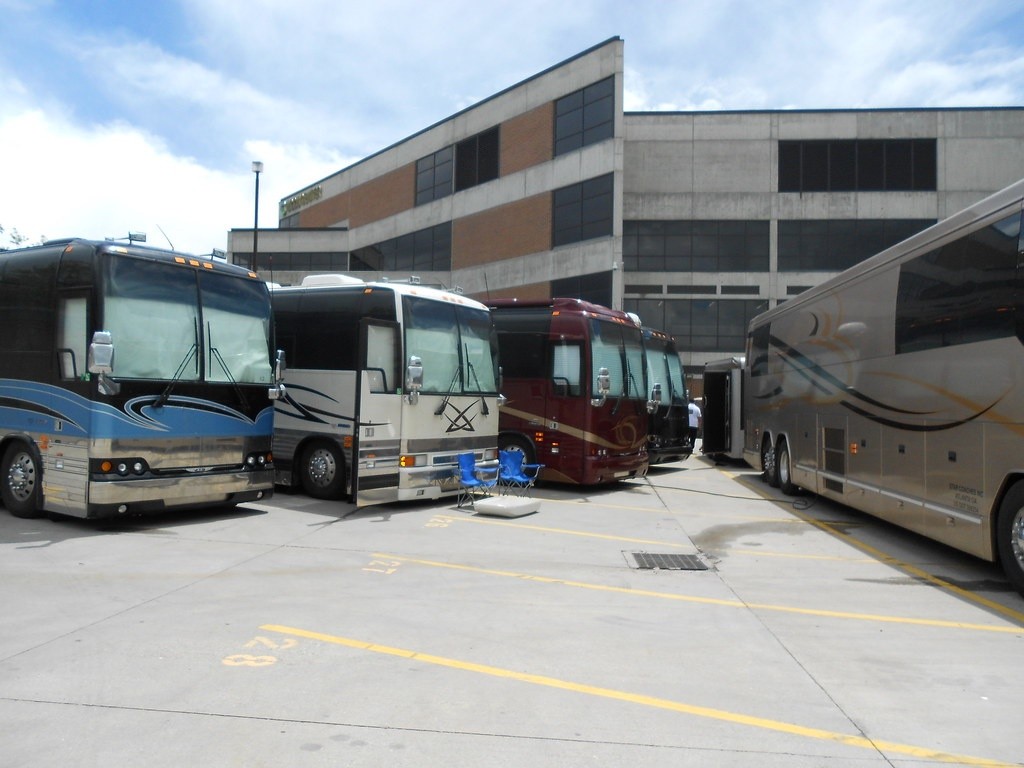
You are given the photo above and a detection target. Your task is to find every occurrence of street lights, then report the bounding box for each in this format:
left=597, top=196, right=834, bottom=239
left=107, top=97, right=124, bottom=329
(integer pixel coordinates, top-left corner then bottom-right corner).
left=251, top=161, right=264, bottom=272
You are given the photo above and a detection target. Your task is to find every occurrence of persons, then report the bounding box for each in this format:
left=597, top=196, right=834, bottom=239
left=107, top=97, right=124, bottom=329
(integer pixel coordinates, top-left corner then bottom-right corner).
left=687, top=399, right=702, bottom=449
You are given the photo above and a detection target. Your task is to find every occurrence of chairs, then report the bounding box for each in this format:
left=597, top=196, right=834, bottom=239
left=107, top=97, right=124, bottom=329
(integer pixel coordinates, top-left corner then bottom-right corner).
left=497, top=450, right=545, bottom=498
left=458, top=452, right=496, bottom=505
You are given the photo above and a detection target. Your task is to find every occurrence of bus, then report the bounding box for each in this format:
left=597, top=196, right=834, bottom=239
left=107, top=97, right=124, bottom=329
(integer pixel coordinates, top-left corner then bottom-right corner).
left=224, top=274, right=508, bottom=508
left=1, top=228, right=289, bottom=522
left=698, top=356, right=745, bottom=459
left=742, top=179, right=1024, bottom=599
left=626, top=311, right=693, bottom=466
left=473, top=296, right=663, bottom=488
